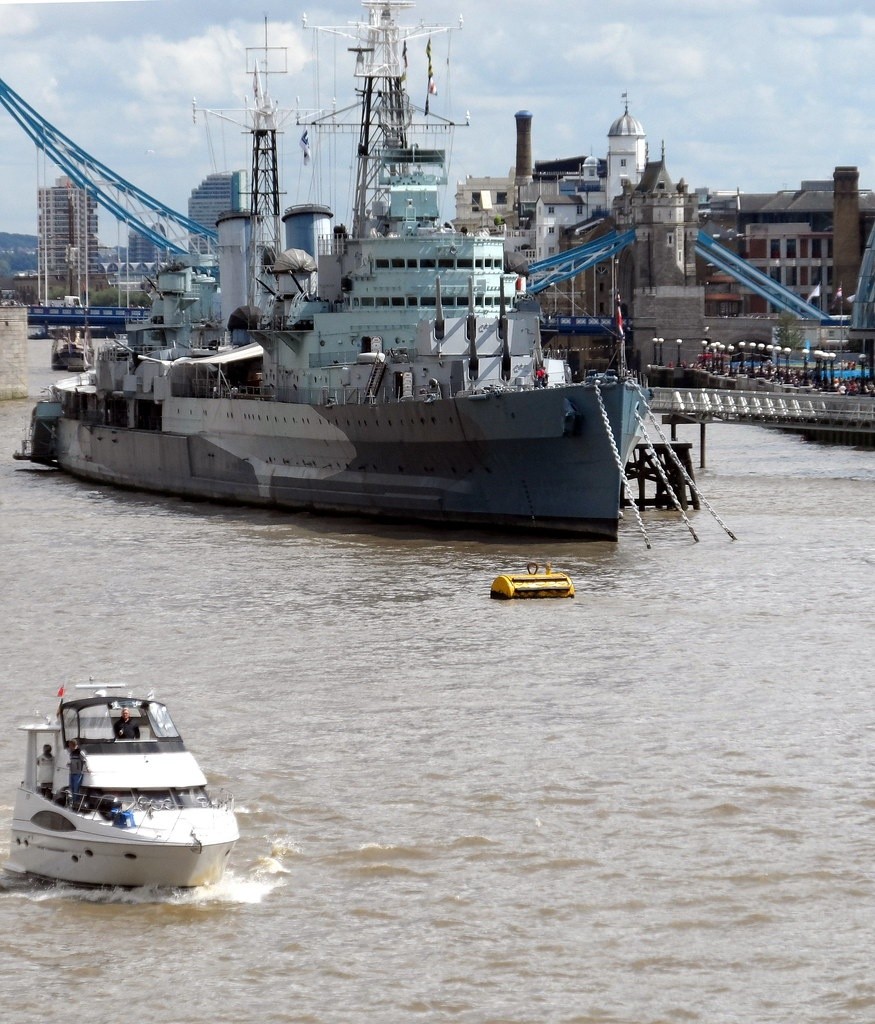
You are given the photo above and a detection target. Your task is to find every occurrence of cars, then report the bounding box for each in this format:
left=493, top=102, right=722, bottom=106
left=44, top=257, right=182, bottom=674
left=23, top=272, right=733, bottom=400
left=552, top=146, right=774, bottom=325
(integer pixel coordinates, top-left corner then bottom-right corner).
left=697, top=351, right=733, bottom=375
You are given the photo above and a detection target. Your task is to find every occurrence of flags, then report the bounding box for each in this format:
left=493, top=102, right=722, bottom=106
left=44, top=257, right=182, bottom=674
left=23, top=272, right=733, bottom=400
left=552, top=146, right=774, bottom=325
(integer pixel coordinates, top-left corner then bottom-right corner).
left=299, top=126, right=310, bottom=166
left=425, top=40, right=437, bottom=116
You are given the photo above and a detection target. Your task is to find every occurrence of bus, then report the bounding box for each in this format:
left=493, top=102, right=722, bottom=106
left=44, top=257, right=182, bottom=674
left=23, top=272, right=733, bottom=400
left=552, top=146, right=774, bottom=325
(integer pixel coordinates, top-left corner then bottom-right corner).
left=729, top=352, right=776, bottom=376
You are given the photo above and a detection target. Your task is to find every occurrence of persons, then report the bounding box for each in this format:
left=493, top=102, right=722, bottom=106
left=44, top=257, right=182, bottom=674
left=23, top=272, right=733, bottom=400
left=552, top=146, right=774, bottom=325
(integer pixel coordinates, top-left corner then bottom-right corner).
left=113, top=709, right=140, bottom=740
left=536, top=365, right=545, bottom=382
left=681, top=360, right=875, bottom=397
left=669, top=360, right=673, bottom=367
left=66, top=739, right=90, bottom=801
left=37, top=744, right=55, bottom=800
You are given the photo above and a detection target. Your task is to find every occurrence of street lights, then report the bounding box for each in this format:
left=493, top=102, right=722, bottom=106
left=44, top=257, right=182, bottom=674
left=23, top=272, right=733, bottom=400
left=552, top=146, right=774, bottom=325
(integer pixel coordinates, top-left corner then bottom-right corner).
left=773, top=345, right=783, bottom=382
left=658, top=337, right=664, bottom=366
left=757, top=343, right=766, bottom=377
left=782, top=347, right=793, bottom=384
left=858, top=353, right=868, bottom=395
left=701, top=340, right=708, bottom=369
left=738, top=341, right=746, bottom=374
left=765, top=344, right=774, bottom=379
left=749, top=342, right=756, bottom=378
left=675, top=338, right=684, bottom=367
left=710, top=341, right=725, bottom=375
left=652, top=337, right=659, bottom=365
left=800, top=348, right=810, bottom=386
left=814, top=349, right=837, bottom=393
left=727, top=345, right=735, bottom=376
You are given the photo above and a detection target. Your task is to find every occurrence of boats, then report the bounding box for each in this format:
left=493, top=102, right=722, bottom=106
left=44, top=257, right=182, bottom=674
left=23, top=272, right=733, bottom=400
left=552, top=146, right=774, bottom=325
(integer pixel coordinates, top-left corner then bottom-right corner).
left=10, top=1, right=652, bottom=543
left=0, top=677, right=241, bottom=891
